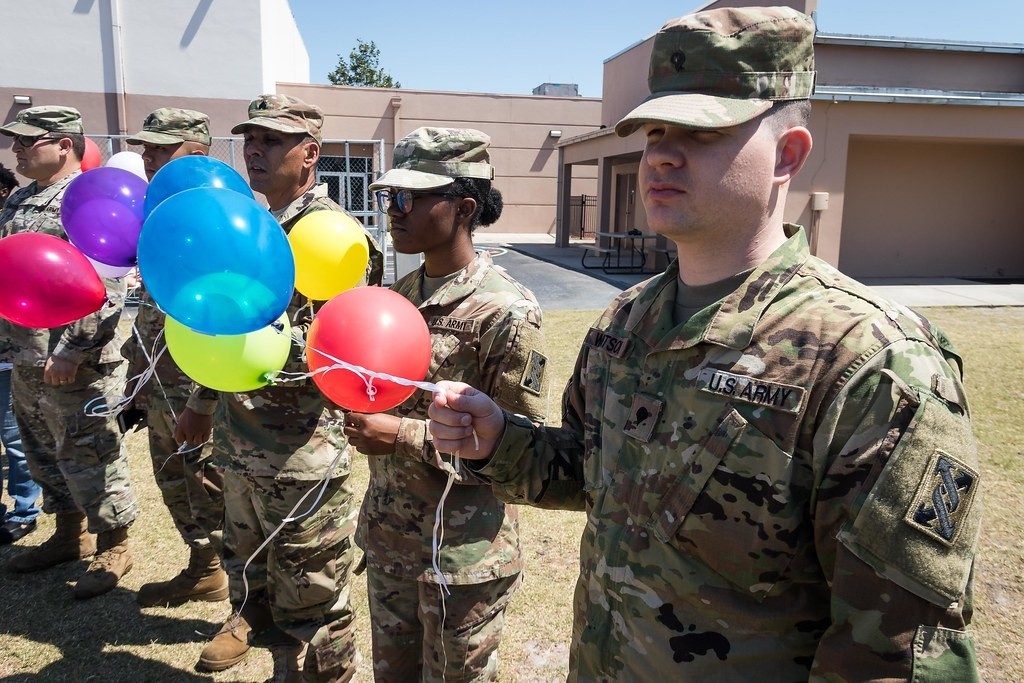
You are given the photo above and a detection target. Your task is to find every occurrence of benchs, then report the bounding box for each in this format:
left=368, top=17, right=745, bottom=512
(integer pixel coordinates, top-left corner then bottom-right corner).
left=579, top=244, right=623, bottom=275
left=633, top=247, right=676, bottom=274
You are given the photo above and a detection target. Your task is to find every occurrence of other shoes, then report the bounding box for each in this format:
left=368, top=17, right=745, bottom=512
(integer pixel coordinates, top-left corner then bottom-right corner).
left=0, top=517, right=37, bottom=544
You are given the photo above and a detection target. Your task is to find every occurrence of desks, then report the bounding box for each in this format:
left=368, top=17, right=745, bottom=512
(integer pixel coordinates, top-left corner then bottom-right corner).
left=596, top=233, right=660, bottom=275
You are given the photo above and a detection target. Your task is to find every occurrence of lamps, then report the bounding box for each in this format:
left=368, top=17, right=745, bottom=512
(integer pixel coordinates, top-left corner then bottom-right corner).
left=13, top=94, right=32, bottom=105
left=550, top=130, right=562, bottom=138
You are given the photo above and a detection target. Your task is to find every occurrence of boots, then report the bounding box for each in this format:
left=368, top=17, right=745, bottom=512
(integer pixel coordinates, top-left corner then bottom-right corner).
left=199, top=613, right=255, bottom=671
left=71, top=528, right=133, bottom=599
left=137, top=547, right=229, bottom=607
left=7, top=515, right=81, bottom=573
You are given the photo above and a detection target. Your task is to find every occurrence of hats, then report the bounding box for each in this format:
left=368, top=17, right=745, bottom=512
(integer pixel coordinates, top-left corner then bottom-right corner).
left=368, top=127, right=495, bottom=192
left=1, top=104, right=83, bottom=137
left=614, top=6, right=817, bottom=138
left=231, top=93, right=324, bottom=148
left=125, top=108, right=213, bottom=147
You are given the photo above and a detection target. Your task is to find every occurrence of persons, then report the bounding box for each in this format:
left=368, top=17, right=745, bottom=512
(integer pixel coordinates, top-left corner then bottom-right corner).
left=0, top=163, right=44, bottom=548
left=427, top=6, right=982, bottom=683
left=115, top=107, right=252, bottom=668
left=318, top=126, right=552, bottom=682
left=0, top=105, right=136, bottom=600
left=212, top=94, right=386, bottom=683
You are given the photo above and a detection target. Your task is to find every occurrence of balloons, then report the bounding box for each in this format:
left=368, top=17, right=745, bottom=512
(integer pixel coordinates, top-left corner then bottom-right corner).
left=136, top=187, right=295, bottom=335
left=143, top=154, right=256, bottom=231
left=0, top=232, right=110, bottom=329
left=61, top=166, right=149, bottom=267
left=286, top=210, right=369, bottom=302
left=164, top=272, right=291, bottom=392
left=105, top=151, right=151, bottom=185
left=68, top=197, right=142, bottom=280
left=80, top=137, right=101, bottom=173
left=304, top=286, right=432, bottom=415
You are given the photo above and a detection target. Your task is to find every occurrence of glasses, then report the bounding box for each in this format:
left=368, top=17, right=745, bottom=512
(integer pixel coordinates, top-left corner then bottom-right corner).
left=13, top=135, right=63, bottom=147
left=375, top=190, right=463, bottom=214
